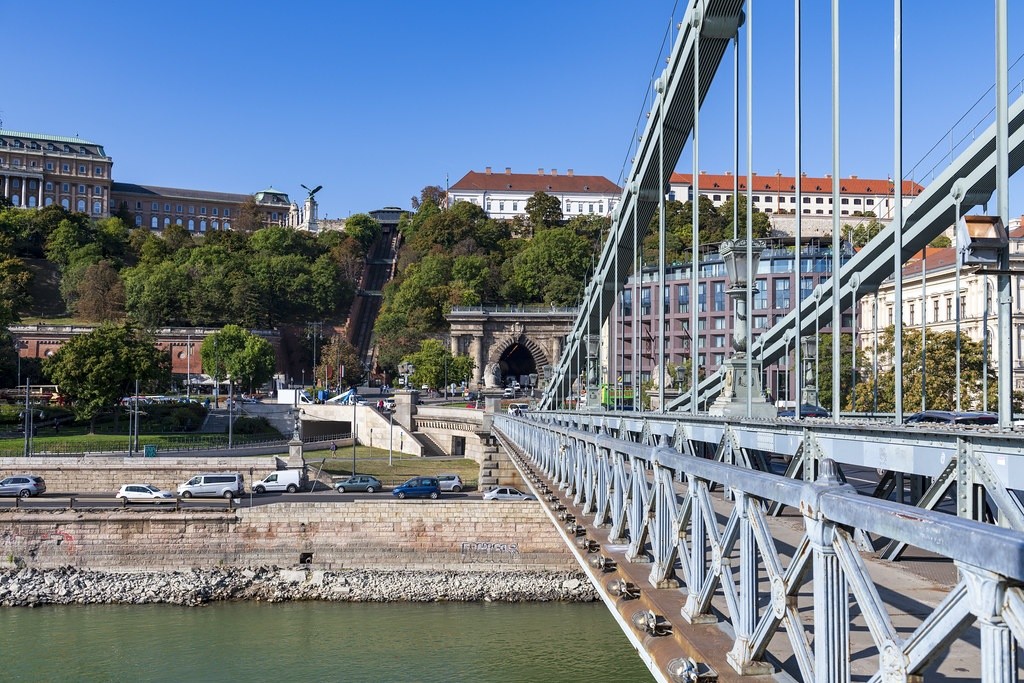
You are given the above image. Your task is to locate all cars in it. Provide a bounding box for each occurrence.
[349,394,371,406]
[508,402,530,416]
[483,486,537,500]
[392,476,441,499]
[334,475,383,493]
[423,474,464,492]
[382,398,396,411]
[778,405,828,418]
[500,374,539,398]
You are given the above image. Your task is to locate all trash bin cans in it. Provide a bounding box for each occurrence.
[144,444,157,457]
[317,390,328,401]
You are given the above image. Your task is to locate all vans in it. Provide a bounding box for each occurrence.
[177,474,246,498]
[250,469,302,493]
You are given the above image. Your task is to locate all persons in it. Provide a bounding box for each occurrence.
[180,369,593,414]
[329,441,338,459]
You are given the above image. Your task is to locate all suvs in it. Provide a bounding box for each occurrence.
[0,475,47,498]
[116,483,173,505]
[877,411,999,478]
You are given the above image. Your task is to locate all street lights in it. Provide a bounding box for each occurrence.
[122,397,153,452]
[20,408,46,456]
